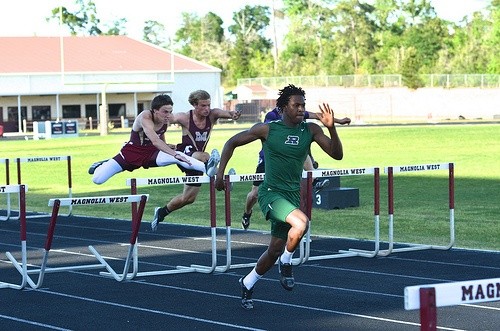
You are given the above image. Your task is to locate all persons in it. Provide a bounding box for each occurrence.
[242,107,351,231]
[214,84,343,310]
[151,90,241,231]
[88,94,221,185]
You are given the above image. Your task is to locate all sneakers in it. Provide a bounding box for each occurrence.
[278,259,296,291]
[204,148,221,179]
[88,158,110,174]
[240,278,255,312]
[227,168,236,191]
[241,208,253,231]
[152,206,166,233]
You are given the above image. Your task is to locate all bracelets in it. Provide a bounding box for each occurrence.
[174,153,181,159]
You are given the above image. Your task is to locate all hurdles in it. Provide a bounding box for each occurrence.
[404,278,500,331]
[191,171,304,273]
[99,175,217,280]
[0,155,73,221]
[292,167,380,264]
[348,162,455,256]
[5,191,150,289]
[0,184,29,289]
